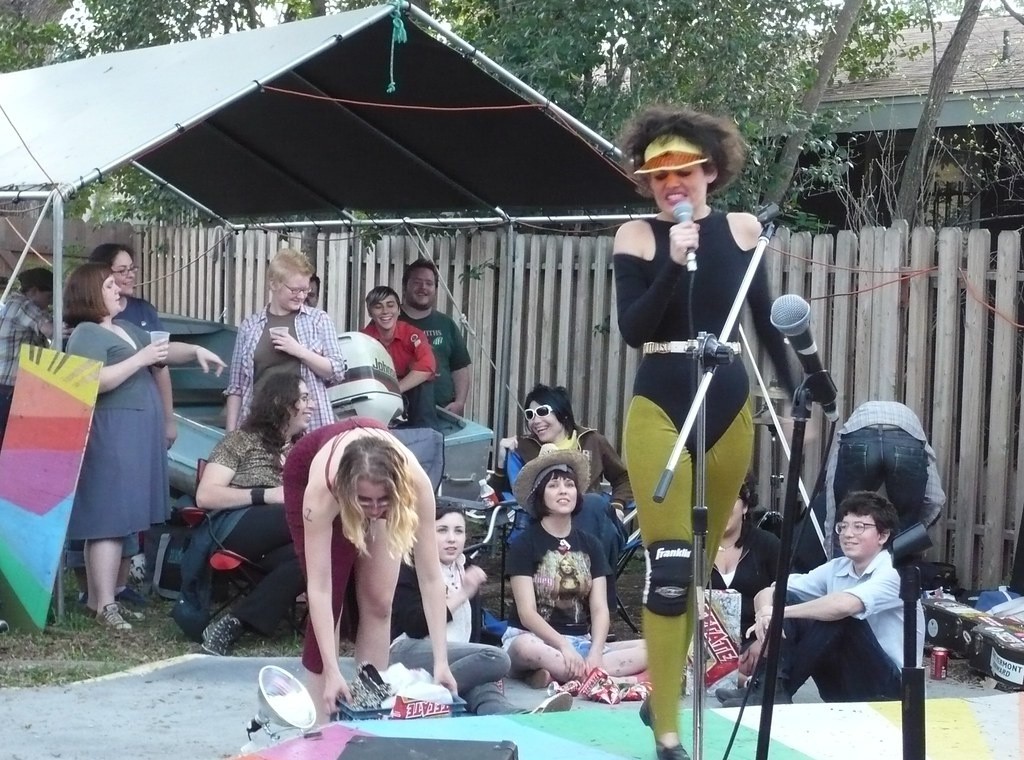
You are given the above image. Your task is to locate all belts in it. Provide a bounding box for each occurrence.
[866,425,900,431]
[643,340,742,355]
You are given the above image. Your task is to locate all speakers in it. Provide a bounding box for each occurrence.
[335,735,520,760]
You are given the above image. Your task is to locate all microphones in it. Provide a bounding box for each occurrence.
[672,200,697,273]
[770,294,840,424]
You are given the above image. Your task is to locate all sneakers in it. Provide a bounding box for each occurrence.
[715,671,792,707]
[201,613,243,657]
[94,603,133,632]
[85,601,146,622]
[115,585,146,605]
[78,592,88,602]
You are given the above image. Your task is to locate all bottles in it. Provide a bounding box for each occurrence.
[479,479,499,507]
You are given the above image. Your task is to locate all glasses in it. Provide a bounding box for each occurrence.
[112,266,138,276]
[358,499,391,508]
[835,521,876,535]
[283,282,313,295]
[436,498,467,515]
[524,404,554,421]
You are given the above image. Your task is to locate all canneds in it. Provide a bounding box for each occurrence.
[931,647,949,679]
[548,667,642,705]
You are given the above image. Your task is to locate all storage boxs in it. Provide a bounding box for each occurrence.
[683,589,742,694]
[331,681,467,723]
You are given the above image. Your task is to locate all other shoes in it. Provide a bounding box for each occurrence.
[529,692,573,713]
[525,669,555,689]
[655,740,690,760]
[638,698,655,732]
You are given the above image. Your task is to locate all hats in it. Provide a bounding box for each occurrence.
[512,444,592,519]
[634,134,712,174]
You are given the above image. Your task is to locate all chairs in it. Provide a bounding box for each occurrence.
[501,446,643,634]
[180,457,308,640]
[388,428,509,527]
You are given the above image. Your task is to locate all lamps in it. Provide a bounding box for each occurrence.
[246,664,317,740]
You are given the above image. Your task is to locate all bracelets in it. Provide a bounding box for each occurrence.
[611,503,623,508]
[251,488,265,506]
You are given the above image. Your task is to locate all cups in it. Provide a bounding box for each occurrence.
[269,326,289,349]
[150,331,170,352]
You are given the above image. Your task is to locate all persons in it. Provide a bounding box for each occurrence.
[64,261,227,631]
[388,507,573,716]
[283,417,458,726]
[0,268,53,452]
[361,286,439,429]
[221,249,345,436]
[716,491,904,708]
[196,372,315,656]
[397,259,472,416]
[517,383,634,641]
[66,242,178,605]
[503,443,648,689]
[705,484,781,688]
[611,108,798,760]
[823,401,946,563]
